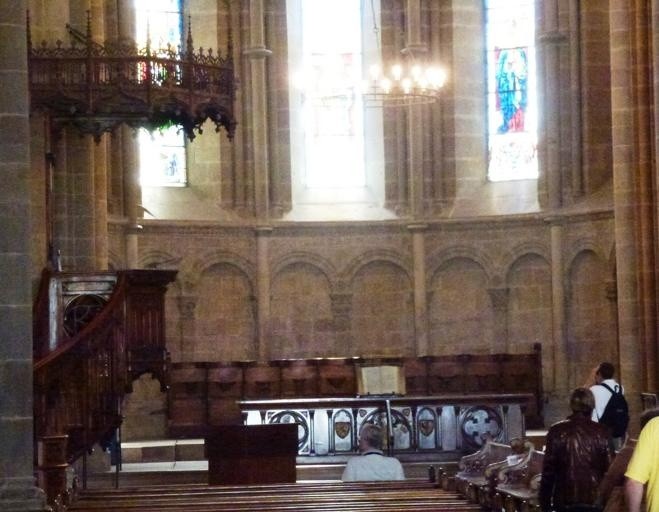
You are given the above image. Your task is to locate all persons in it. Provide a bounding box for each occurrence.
[538,387,616,512]
[341,424,406,480]
[640,408,659,429]
[583,362,628,454]
[623,416,659,512]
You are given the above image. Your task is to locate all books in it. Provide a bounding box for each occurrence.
[356,364,407,395]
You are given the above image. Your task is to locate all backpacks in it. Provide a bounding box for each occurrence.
[598,385,630,440]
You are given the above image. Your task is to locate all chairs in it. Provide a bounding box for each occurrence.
[456,434,545,509]
